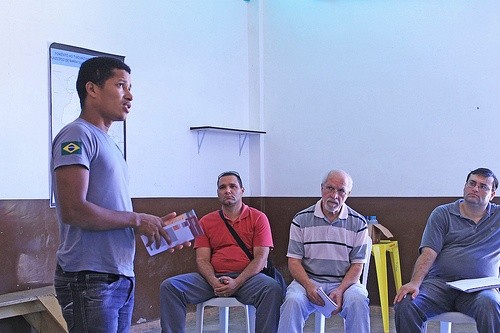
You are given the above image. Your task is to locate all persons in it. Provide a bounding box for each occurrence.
[160,171,283,333]
[50,57,191,333]
[278,170,371,333]
[394,168,500,333]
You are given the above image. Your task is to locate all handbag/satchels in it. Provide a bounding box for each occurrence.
[260,256,276,279]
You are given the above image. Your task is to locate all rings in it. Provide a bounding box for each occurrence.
[223,293,225,297]
[219,277,221,281]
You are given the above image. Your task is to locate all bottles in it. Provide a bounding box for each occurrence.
[364,216,369,225]
[369,216,378,224]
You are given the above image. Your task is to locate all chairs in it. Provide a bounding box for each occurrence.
[313,237,372,333]
[196,295,256,333]
[421,310,478,333]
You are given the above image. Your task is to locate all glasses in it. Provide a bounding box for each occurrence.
[218,171,240,179]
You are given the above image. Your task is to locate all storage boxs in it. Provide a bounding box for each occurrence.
[369,224,393,243]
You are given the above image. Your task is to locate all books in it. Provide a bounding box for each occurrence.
[310,287,339,319]
[445,277,500,293]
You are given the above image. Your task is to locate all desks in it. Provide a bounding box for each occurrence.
[371,240,403,333]
